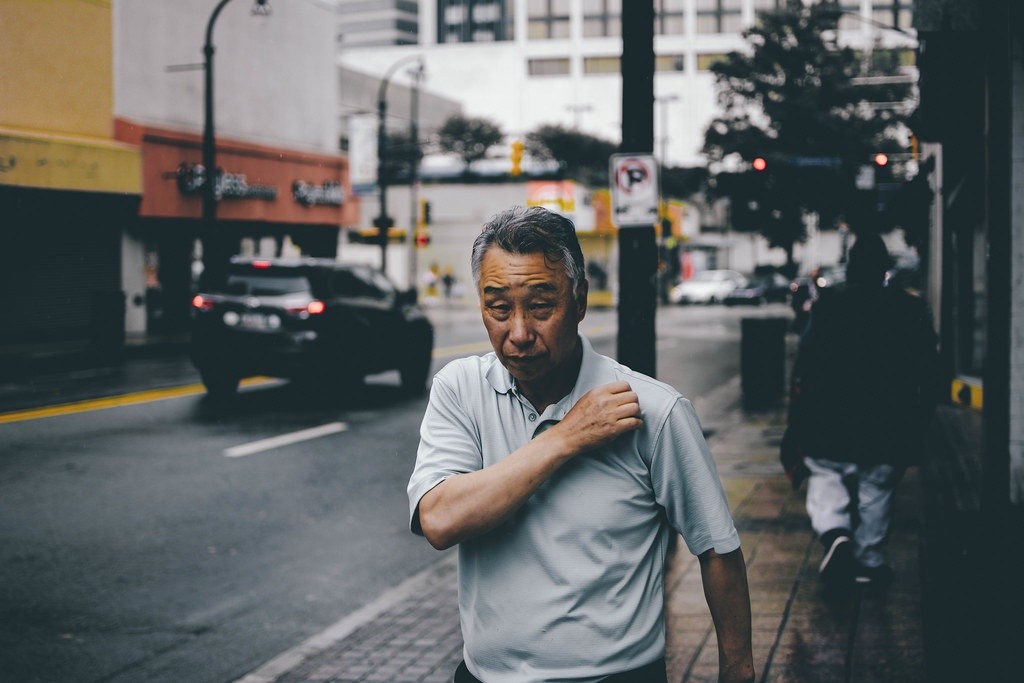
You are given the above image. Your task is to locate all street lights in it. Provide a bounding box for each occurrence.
[201,0,274,270]
[375,52,426,270]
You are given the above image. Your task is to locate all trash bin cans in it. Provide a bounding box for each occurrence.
[740,314,786,406]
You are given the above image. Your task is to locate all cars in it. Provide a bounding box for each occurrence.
[729,264,846,306]
[667,269,749,306]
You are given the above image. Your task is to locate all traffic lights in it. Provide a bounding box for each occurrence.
[875,153,888,166]
[753,156,766,171]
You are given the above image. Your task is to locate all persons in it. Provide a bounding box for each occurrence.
[782,242,941,583]
[407,206,756,683]
[792,266,821,333]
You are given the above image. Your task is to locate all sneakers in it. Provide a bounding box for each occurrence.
[818,526,854,579]
[853,568,873,584]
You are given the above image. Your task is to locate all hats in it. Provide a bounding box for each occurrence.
[848,234,896,272]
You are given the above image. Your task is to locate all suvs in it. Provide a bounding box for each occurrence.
[183,256,437,415]
